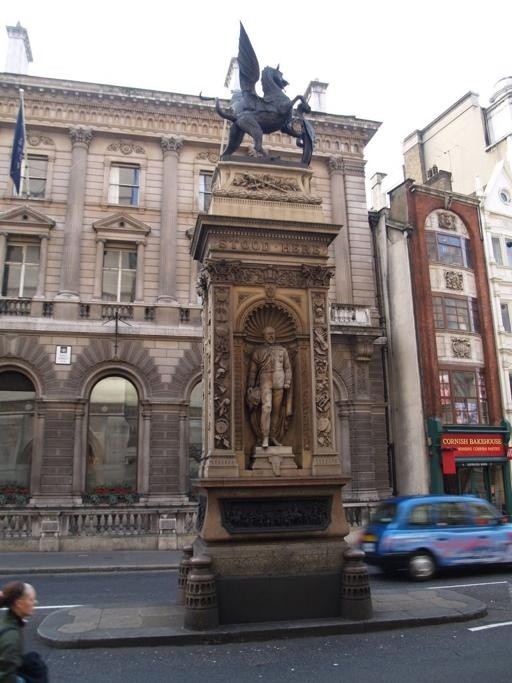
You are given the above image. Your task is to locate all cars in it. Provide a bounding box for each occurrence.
[358,492,512,583]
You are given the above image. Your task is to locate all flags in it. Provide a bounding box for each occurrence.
[10,100,24,193]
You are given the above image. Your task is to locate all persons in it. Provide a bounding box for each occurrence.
[249,325,293,448]
[0,580,49,683]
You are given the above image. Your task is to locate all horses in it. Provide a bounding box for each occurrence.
[199,61,311,158]
